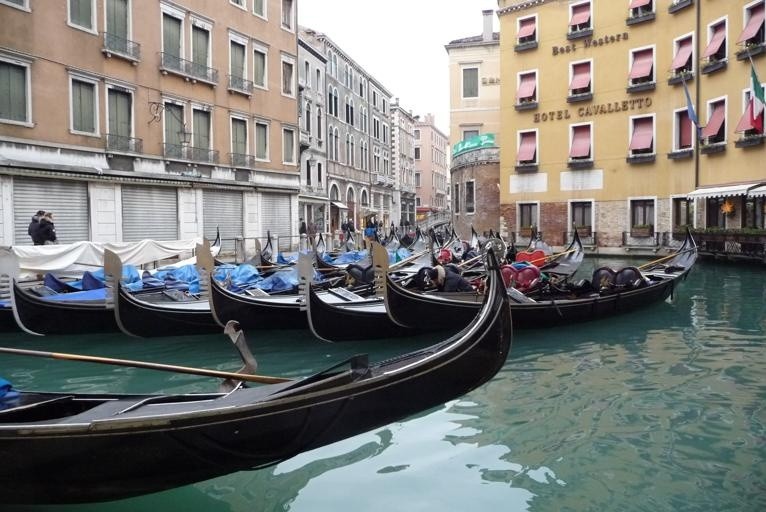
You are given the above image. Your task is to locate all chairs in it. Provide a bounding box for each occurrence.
[347,240,642,297]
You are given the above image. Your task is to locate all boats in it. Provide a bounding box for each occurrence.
[0,248,515,508]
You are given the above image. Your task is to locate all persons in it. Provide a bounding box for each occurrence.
[300,213,410,247]
[38,213,56,245]
[415,266,474,292]
[28,210,45,245]
